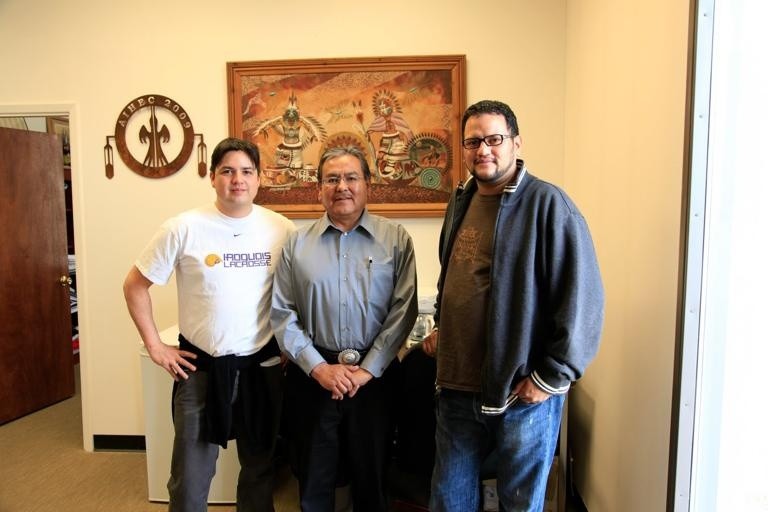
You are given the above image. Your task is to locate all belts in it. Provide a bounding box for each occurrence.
[313,346,368,365]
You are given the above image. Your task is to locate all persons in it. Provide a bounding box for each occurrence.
[269,145,419,508]
[122,135,294,512]
[421,100,604,511]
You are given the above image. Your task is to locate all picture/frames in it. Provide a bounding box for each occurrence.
[224,52,468,221]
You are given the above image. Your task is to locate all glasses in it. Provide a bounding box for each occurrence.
[462,134,515,149]
